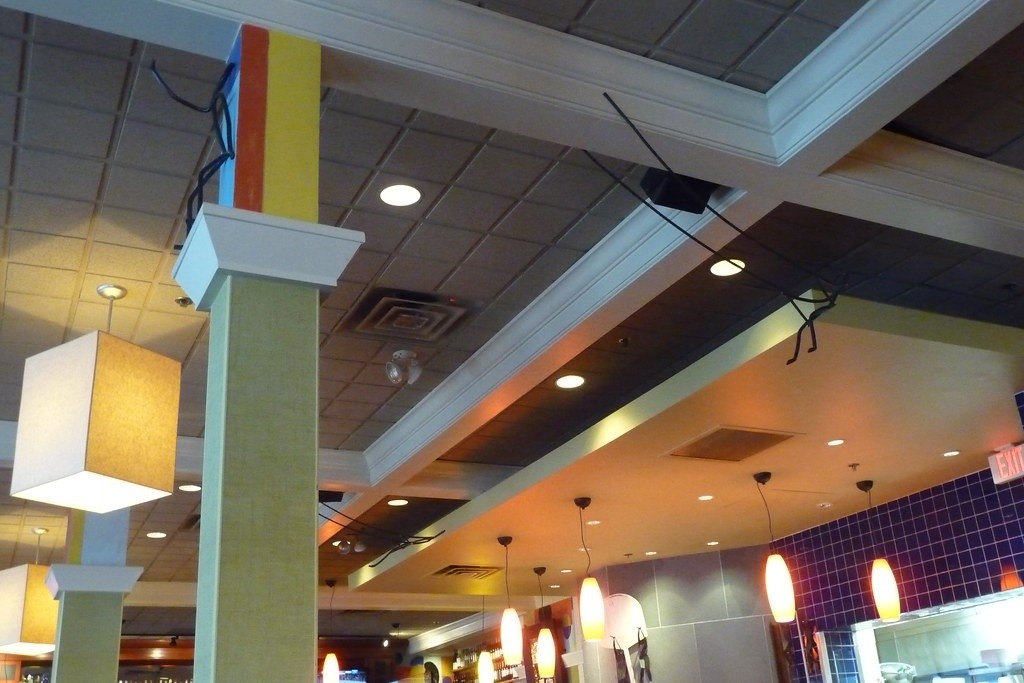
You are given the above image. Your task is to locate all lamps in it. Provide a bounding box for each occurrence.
[497,535,523,665]
[856,480,900,623]
[533,566,556,678]
[574,496,605,642]
[7,285,182,514]
[477,595,495,683]
[323,579,340,683]
[752,471,795,623]
[0,528,60,656]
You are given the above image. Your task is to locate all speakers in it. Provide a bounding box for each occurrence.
[640,168,719,215]
